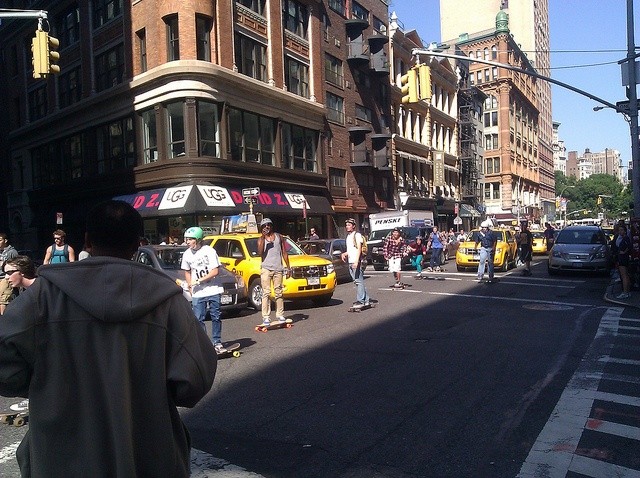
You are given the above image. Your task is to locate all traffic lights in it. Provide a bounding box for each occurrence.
[31,30,60,79]
[396,64,433,104]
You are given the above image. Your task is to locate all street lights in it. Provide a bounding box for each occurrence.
[516,166,538,226]
[559,185,575,220]
[592,103,632,128]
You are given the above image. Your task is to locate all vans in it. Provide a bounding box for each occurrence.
[455,227,518,272]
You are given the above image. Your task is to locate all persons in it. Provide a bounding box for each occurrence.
[543,224,556,253]
[383,226,406,288]
[518,220,534,276]
[426,226,446,271]
[440,227,468,245]
[341,217,370,308]
[609,219,633,299]
[307,228,319,239]
[406,235,426,279]
[255,218,291,323]
[474,221,497,282]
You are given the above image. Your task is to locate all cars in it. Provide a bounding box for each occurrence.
[531,230,548,254]
[548,225,609,273]
[137,245,240,316]
[296,239,368,284]
[443,234,460,260]
[203,233,337,311]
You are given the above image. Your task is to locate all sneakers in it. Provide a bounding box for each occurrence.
[214,344,227,352]
[263,317,271,325]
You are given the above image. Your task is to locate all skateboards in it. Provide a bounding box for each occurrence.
[393,285,407,291]
[349,302,376,312]
[472,276,498,285]
[435,268,448,273]
[0,408,30,427]
[256,318,292,332]
[215,343,240,358]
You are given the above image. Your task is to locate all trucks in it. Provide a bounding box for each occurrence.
[365,209,436,271]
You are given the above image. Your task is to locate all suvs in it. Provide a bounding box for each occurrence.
[384,225,445,270]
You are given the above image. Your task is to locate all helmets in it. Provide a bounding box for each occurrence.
[183,227,203,243]
[260,218,274,228]
[345,218,356,224]
